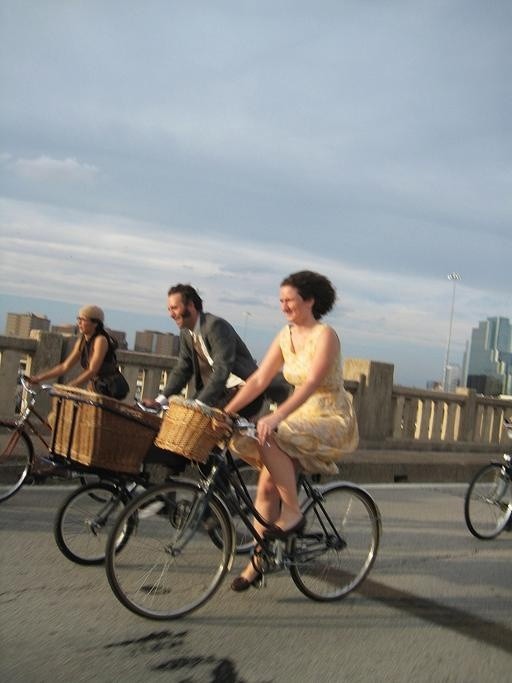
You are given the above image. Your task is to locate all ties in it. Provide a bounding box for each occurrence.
[191,335,214,388]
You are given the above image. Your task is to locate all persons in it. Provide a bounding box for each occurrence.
[138,284,265,530]
[221,270,360,593]
[30,304,129,466]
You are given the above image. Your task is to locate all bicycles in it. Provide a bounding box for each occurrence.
[465,417,512,539]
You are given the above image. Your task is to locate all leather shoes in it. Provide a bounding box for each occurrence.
[230,568,263,593]
[262,512,307,541]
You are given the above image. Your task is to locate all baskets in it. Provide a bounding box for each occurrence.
[49,384,163,476]
[153,393,233,464]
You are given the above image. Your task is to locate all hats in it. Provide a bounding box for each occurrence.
[80,305,103,320]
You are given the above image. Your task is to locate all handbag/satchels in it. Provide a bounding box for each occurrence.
[92,371,129,400]
